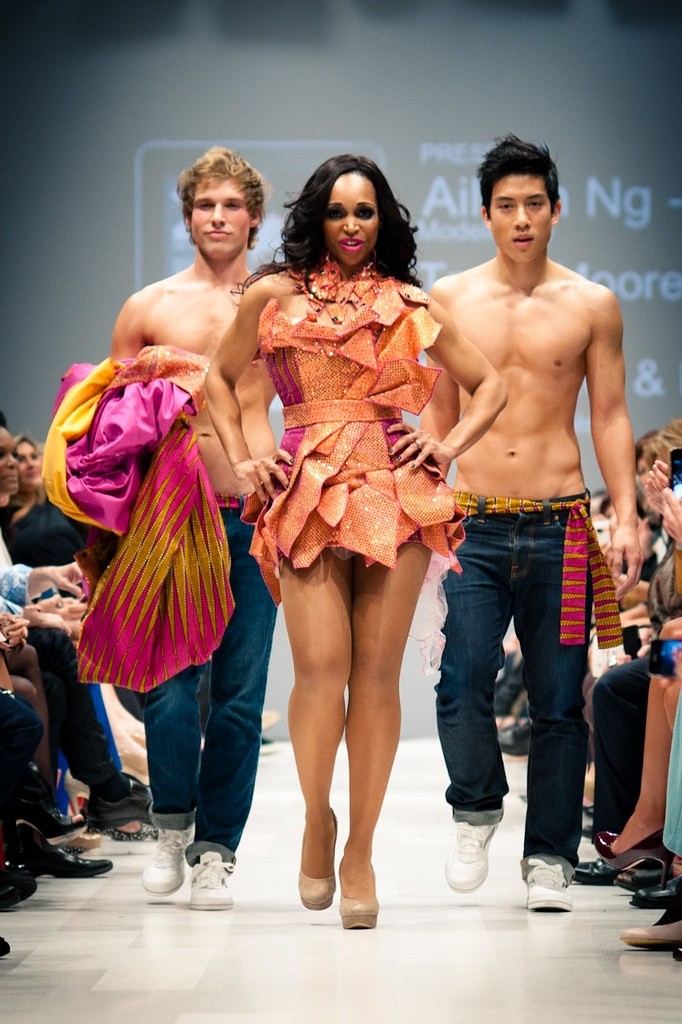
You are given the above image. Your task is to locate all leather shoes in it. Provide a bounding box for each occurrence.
[0,867,36,905]
[85,772,154,828]
[23,844,114,879]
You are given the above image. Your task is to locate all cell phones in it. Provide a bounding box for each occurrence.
[648,639,682,678]
[669,448,682,500]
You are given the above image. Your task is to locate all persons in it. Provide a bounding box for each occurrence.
[496,417,682,961]
[205,155,507,931]
[0,409,281,958]
[112,147,278,909]
[420,134,644,910]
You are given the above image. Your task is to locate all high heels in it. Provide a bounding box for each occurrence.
[296,806,338,909]
[85,819,160,842]
[10,797,84,838]
[337,857,378,930]
[594,826,675,889]
[59,843,86,855]
[64,768,94,817]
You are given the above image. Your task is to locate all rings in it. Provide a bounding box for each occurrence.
[415,439,421,449]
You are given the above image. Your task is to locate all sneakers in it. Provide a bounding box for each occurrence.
[141,828,191,896]
[189,850,235,911]
[444,823,498,894]
[525,858,573,913]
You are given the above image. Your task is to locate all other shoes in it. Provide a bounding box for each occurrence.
[499,722,531,755]
[573,856,682,960]
